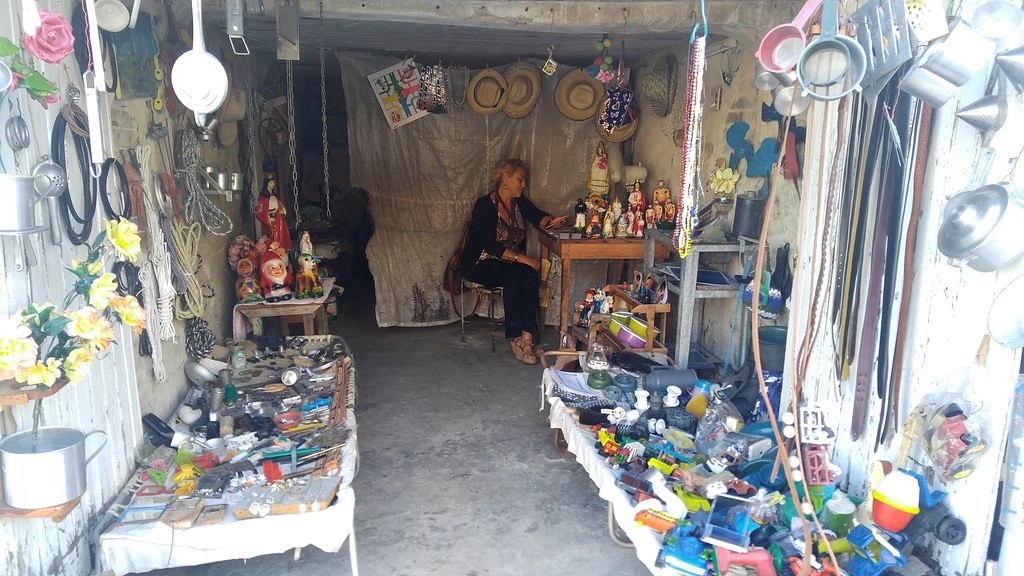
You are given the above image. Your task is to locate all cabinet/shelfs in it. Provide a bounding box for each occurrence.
[641,226,767,376]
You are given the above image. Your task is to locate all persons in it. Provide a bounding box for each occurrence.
[255,171,291,248]
[586,140,610,197]
[574,195,645,238]
[260,251,293,302]
[653,199,663,223]
[645,204,655,229]
[654,179,670,208]
[460,159,570,365]
[628,179,647,213]
[664,198,675,223]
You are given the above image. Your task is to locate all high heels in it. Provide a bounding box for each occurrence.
[511,340,543,358]
[516,339,536,364]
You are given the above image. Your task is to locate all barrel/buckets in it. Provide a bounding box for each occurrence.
[748,325,787,373]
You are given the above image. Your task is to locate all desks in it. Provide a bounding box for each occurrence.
[231,275,335,338]
[532,221,673,355]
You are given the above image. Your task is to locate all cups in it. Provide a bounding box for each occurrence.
[641,364,700,395]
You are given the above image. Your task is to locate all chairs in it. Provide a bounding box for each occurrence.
[455,220,504,355]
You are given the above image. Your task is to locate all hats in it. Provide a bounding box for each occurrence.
[596,88,639,142]
[553,68,605,120]
[465,67,508,115]
[501,62,543,119]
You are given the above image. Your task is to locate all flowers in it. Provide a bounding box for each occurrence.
[0,216,148,453]
[0,7,77,111]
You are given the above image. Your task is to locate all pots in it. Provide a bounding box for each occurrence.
[913,18,997,87]
[936,179,1024,273]
[229,173,245,190]
[209,171,229,190]
[897,59,956,110]
[0,425,109,509]
[200,166,218,190]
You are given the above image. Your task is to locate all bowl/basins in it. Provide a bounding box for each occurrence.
[617,326,646,348]
[609,319,628,334]
[629,315,661,341]
[611,311,633,325]
